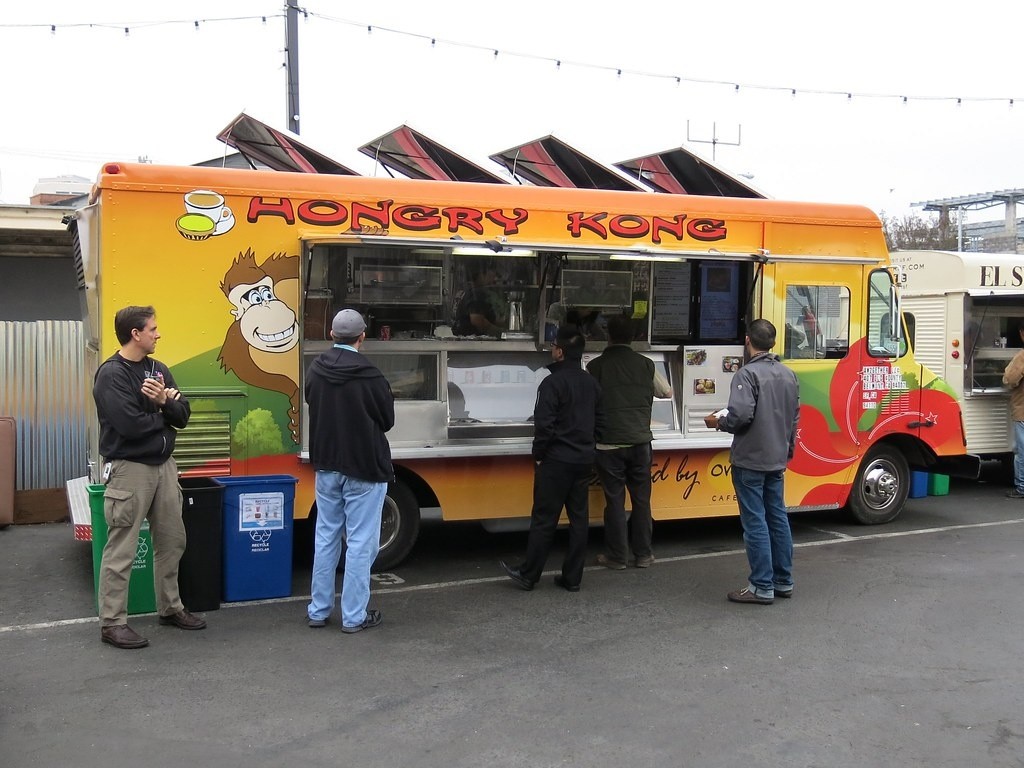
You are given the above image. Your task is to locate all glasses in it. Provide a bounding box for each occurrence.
[550,341,559,348]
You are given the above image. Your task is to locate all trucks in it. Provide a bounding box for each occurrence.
[842,249,1024,477]
[87,161,981,572]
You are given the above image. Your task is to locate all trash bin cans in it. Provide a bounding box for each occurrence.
[212,474,299,601]
[176,476,226,612]
[84,484,157,615]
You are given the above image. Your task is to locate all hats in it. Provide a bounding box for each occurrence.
[331,308,367,339]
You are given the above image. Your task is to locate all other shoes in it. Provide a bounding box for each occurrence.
[1005,489,1024,498]
[341,609,381,633]
[727,587,774,605]
[159,608,207,629]
[500,561,534,591]
[553,574,580,591]
[773,589,793,598]
[635,554,655,567]
[304,614,332,626]
[101,624,150,649]
[597,554,626,569]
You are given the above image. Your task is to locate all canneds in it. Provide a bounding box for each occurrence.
[381,325,390,342]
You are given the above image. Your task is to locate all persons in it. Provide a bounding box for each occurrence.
[454,255,642,342]
[305,309,395,633]
[704,319,799,604]
[585,315,672,571]
[92,305,206,649]
[1003,325,1024,499]
[501,328,606,592]
